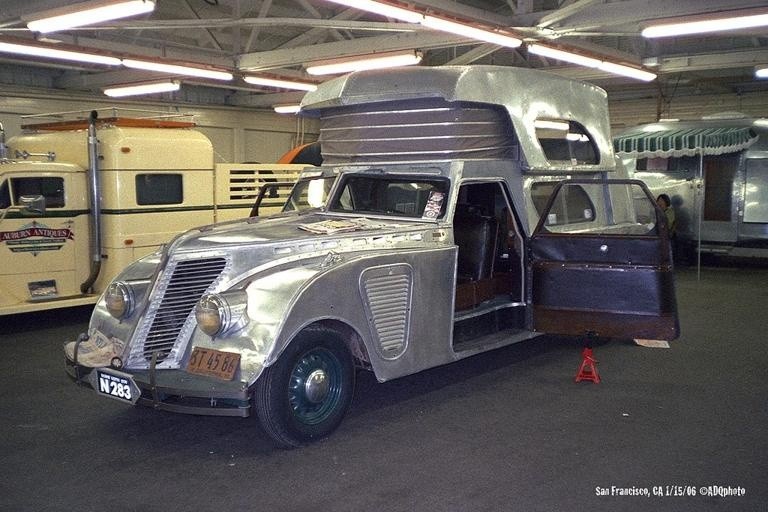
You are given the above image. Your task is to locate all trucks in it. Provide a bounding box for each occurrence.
[0,106,334,324]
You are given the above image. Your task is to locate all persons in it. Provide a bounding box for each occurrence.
[650,194,676,238]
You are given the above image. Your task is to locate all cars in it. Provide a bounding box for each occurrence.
[61,62,684,452]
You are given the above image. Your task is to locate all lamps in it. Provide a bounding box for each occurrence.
[1,0,768,116]
